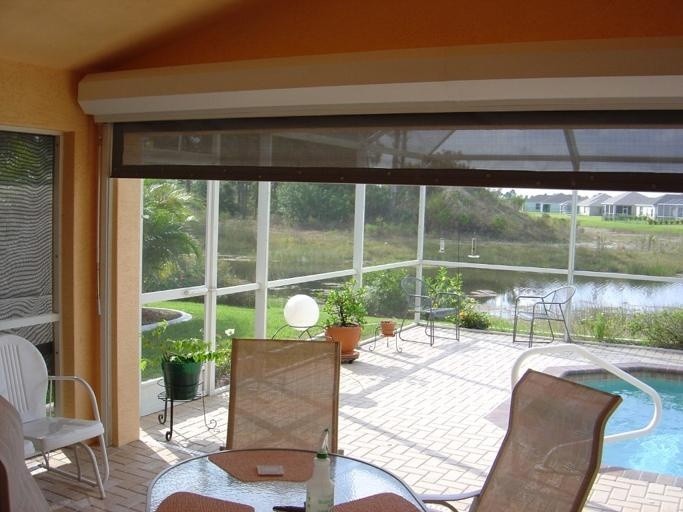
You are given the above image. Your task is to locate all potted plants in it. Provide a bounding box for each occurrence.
[138,318,233,401]
[380,319,395,336]
[323,278,368,354]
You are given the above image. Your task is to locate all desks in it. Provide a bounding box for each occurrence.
[146,449,428,511]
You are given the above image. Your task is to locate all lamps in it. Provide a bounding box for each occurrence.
[271,292,320,340]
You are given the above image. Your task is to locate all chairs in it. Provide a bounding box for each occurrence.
[416,368,623,512]
[220,337,344,457]
[512,286,576,348]
[399,273,460,347]
[0,333,110,512]
